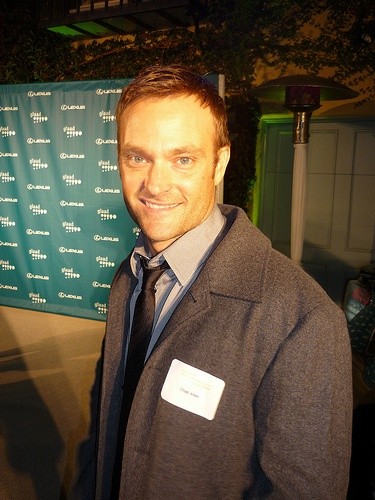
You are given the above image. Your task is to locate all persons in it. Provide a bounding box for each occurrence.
[94,63,353,500]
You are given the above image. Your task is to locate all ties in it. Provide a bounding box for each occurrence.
[111,256,171,500]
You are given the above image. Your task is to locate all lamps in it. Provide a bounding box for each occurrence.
[251,73,359,143]
